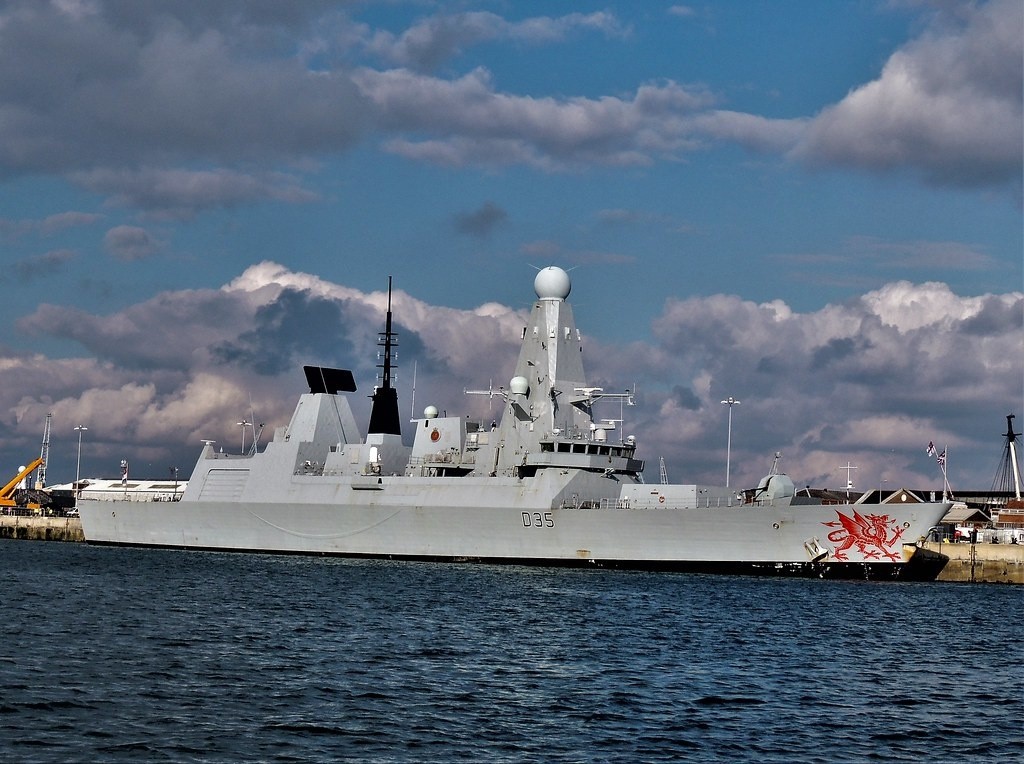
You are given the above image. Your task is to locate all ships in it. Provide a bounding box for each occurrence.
[74,263,957,586]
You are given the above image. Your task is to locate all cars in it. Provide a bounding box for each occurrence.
[66,507,79,518]
